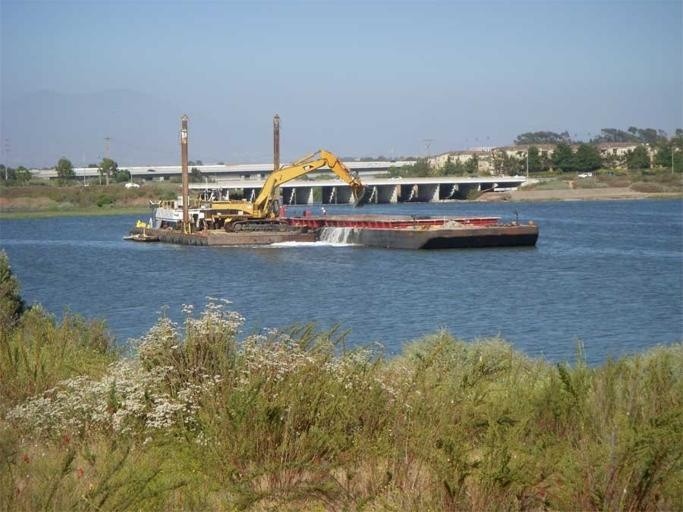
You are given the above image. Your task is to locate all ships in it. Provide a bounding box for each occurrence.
[123,113,321,245]
[288,216,539,249]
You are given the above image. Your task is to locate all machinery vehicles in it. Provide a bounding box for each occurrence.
[204,150,364,232]
